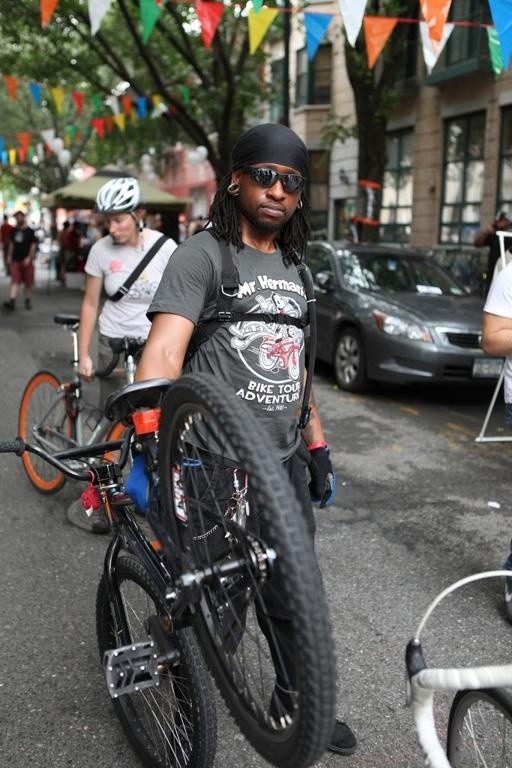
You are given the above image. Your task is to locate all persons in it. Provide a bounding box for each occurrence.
[475,217,512,296]
[77,176,177,418]
[59,221,71,286]
[3,211,39,310]
[135,124,357,756]
[73,219,81,250]
[478,256,511,618]
[0,215,15,275]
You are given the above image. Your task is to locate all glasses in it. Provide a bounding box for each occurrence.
[240,166,305,192]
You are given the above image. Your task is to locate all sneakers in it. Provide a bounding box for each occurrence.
[266,704,357,755]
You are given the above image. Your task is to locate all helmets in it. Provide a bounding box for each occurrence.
[96,177,140,214]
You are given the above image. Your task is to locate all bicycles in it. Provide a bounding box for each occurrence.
[49,380,335,760]
[7,311,178,502]
[446,687,512,767]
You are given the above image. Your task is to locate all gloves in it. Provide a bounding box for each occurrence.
[308,446,337,508]
[125,455,160,517]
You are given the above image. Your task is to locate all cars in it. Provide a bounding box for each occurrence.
[292,240,496,397]
